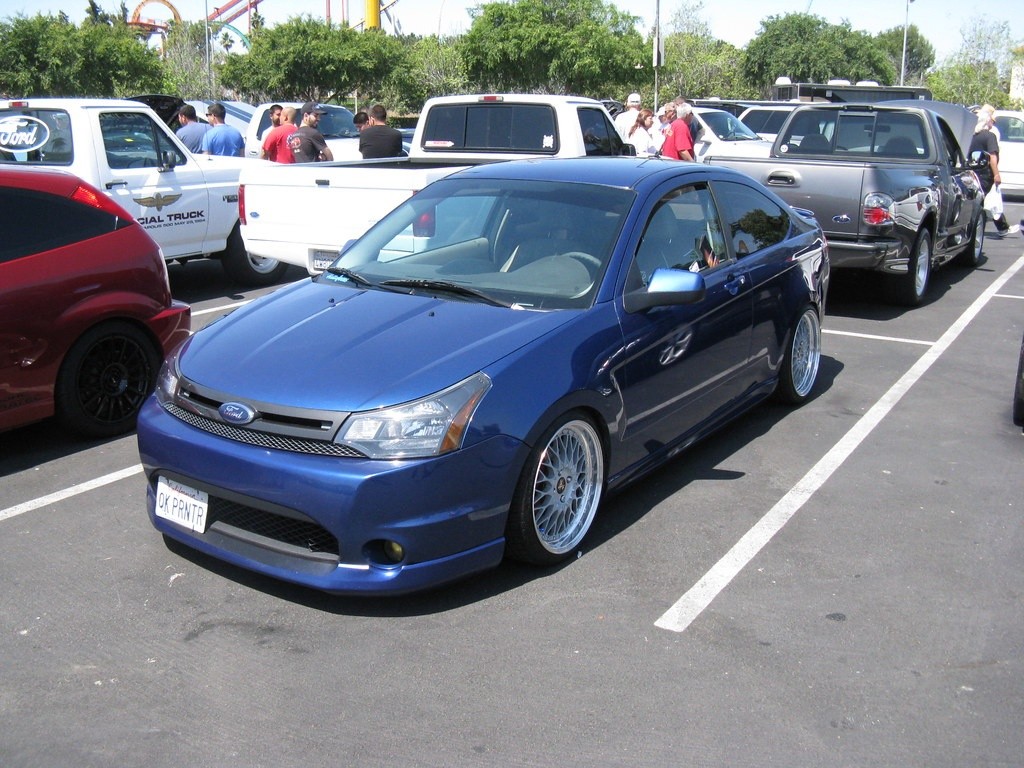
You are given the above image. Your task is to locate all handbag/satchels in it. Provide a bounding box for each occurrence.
[983,183,1003,220]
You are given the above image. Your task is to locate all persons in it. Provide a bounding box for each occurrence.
[966,103,1019,236]
[202,103,246,157]
[353,112,372,153]
[359,104,402,159]
[176,104,213,154]
[615,92,695,162]
[287,101,334,163]
[260,104,297,160]
[261,106,299,163]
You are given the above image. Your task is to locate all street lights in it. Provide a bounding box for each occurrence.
[900,0,916,86]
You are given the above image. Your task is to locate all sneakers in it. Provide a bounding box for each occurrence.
[999,224,1020,236]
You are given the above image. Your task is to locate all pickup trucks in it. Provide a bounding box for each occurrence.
[0,94,637,286]
[684,77,1024,306]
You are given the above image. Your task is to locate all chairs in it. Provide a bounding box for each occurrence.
[883,136,918,155]
[499,199,583,273]
[635,201,680,282]
[798,133,831,154]
[565,198,618,280]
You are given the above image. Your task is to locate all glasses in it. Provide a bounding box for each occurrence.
[206,112,215,117]
[689,113,694,116]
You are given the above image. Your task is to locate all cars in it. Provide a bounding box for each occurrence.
[137,156,830,599]
[0,165,191,438]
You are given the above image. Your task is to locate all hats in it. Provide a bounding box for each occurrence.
[657,106,666,118]
[628,93,641,105]
[973,104,996,124]
[301,102,328,116]
